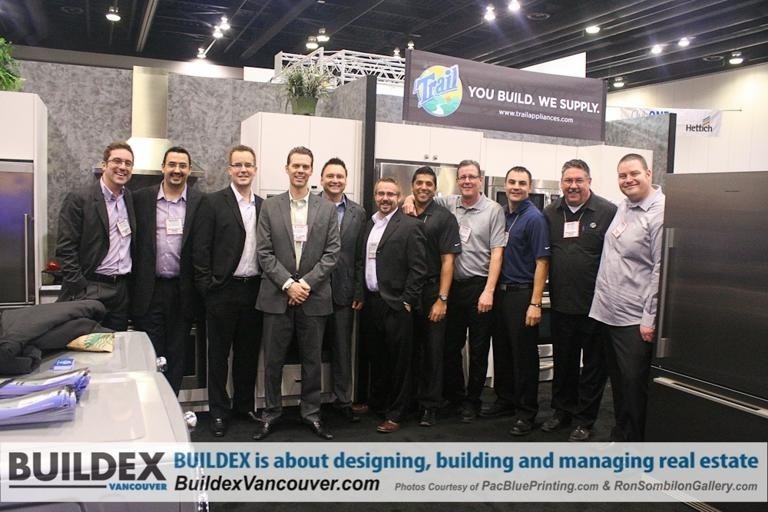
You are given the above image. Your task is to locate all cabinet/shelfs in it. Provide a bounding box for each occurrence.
[485,136,521,179]
[374,121,483,166]
[240,111,360,200]
[579,145,653,206]
[555,145,578,183]
[521,142,557,181]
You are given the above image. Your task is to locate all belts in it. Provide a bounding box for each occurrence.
[497,282,531,292]
[366,290,381,299]
[92,273,124,284]
[232,275,260,286]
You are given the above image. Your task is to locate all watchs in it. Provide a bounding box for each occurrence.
[528,300,544,309]
[437,292,448,300]
[283,280,295,291]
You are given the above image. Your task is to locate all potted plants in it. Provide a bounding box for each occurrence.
[270,64,335,116]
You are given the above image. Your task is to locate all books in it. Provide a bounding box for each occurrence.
[0,367,92,403]
[0,387,76,427]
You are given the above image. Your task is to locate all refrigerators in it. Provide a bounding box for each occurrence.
[644,170,768,446]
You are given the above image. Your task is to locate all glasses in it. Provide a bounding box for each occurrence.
[457,175,482,181]
[163,162,188,169]
[105,157,133,166]
[375,191,398,198]
[229,162,256,169]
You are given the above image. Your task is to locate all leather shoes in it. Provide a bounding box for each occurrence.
[301,416,336,440]
[509,416,536,436]
[350,400,370,414]
[239,411,265,426]
[418,406,438,427]
[459,402,478,422]
[376,419,400,433]
[212,416,225,437]
[480,403,515,418]
[252,419,277,440]
[539,418,563,433]
[567,425,593,442]
[341,406,363,423]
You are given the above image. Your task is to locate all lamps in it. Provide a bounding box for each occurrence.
[679,38,691,48]
[393,47,401,59]
[484,4,497,22]
[651,45,662,56]
[196,45,208,62]
[106,0,122,23]
[407,41,415,50]
[317,28,330,43]
[218,15,230,32]
[212,24,223,41]
[509,0,520,11]
[614,76,625,88]
[728,52,744,64]
[305,34,319,50]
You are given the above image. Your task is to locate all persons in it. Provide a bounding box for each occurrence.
[131,146,208,396]
[193,144,265,438]
[588,154,666,441]
[543,158,620,432]
[478,166,552,436]
[352,177,429,432]
[315,157,369,422]
[402,161,506,424]
[397,166,463,425]
[253,147,343,439]
[56,143,138,331]
